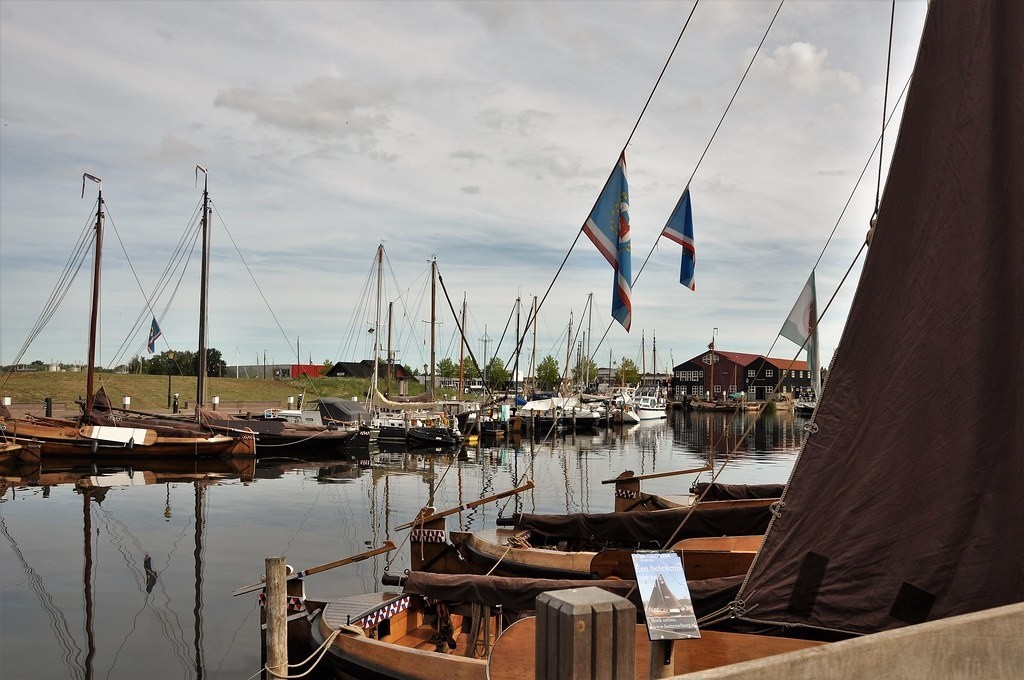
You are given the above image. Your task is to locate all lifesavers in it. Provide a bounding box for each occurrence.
[556,410,561,418]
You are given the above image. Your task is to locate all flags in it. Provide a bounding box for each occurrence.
[583,149,632,334]
[147,318,162,354]
[780,271,821,400]
[661,186,696,291]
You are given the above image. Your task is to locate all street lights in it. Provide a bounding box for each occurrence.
[423,364,429,393]
[166,349,174,410]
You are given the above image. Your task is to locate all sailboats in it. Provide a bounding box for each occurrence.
[1,0,1024,680]
